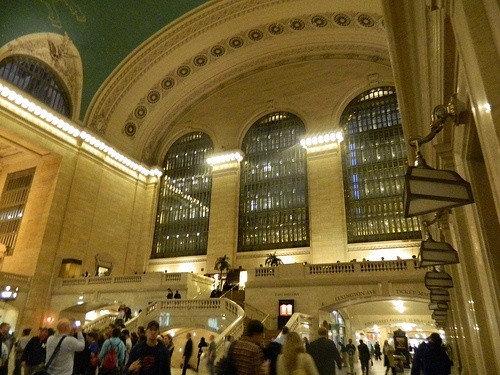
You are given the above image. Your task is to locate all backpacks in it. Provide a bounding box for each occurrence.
[216,340,239,375]
[103,338,121,369]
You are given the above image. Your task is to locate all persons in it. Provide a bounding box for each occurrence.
[345,338,356,373]
[0,323,11,375]
[80,271,100,278]
[198,337,207,371]
[370,342,382,360]
[209,335,219,375]
[12,318,174,375]
[209,289,222,298]
[382,341,397,375]
[222,320,342,375]
[337,341,348,367]
[166,288,173,300]
[410,333,454,375]
[174,290,181,299]
[183,333,196,375]
[116,305,133,318]
[358,340,371,375]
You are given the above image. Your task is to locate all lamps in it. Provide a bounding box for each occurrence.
[424,265,453,329]
[416,209,459,268]
[401,95,474,218]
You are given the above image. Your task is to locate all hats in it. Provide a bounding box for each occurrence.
[146,320,159,329]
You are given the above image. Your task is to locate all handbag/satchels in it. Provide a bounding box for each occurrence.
[31,364,50,375]
